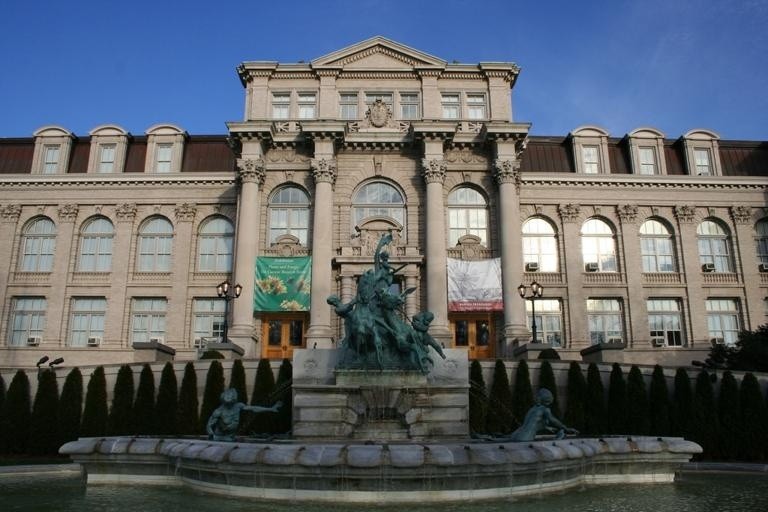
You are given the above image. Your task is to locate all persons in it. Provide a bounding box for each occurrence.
[508,387,580,442]
[326,232,446,376]
[206,386,283,441]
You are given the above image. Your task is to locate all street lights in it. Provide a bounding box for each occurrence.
[216,279,244,341]
[519,279,546,344]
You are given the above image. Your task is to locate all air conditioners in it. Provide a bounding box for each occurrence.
[150,337,163,345]
[526,262,768,346]
[27,335,41,346]
[194,336,209,346]
[87,336,100,346]
[698,171,710,176]
